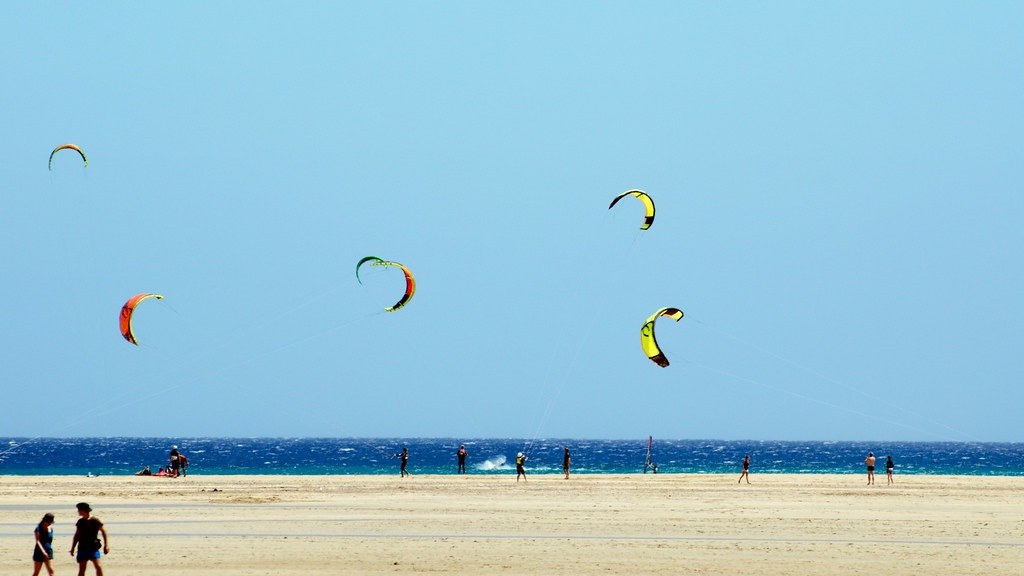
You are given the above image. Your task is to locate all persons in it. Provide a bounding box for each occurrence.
[864,451,875,486]
[140,445,189,478]
[884,455,895,486]
[515,452,529,482]
[457,445,468,475]
[71,502,110,576]
[32,513,57,576]
[562,448,571,479]
[738,456,752,484]
[393,448,410,478]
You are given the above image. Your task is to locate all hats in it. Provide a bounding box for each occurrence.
[43,512,55,523]
[517,452,523,457]
[172,445,178,449]
[76,502,93,511]
